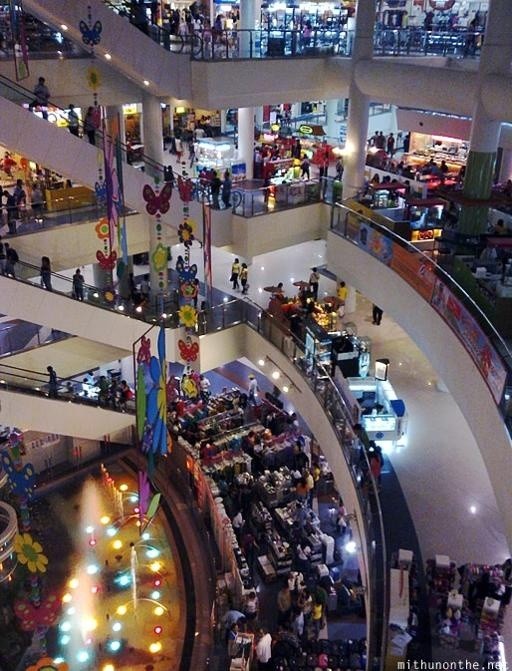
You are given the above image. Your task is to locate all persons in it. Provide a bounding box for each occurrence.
[198,373,211,401]
[3,242,20,279]
[66,370,129,412]
[3,189,16,235]
[277,283,283,292]
[0,235,3,256]
[40,256,52,290]
[247,374,261,407]
[171,419,384,671]
[193,279,199,307]
[18,190,26,217]
[480,240,497,259]
[188,137,196,169]
[318,153,329,182]
[140,274,151,300]
[337,281,348,318]
[29,76,51,120]
[214,15,222,30]
[222,171,232,209]
[72,268,85,301]
[47,366,58,398]
[0,151,10,166]
[177,17,188,36]
[67,104,79,137]
[240,263,248,294]
[372,303,383,326]
[231,16,237,38]
[369,129,466,184]
[309,267,320,301]
[31,183,43,218]
[200,167,207,177]
[494,219,507,235]
[126,272,137,300]
[230,393,247,410]
[83,107,96,145]
[253,141,281,179]
[3,155,17,177]
[13,179,22,219]
[284,104,291,124]
[333,156,344,181]
[231,257,241,290]
[193,124,207,138]
[203,19,211,42]
[263,170,272,202]
[174,134,183,163]
[303,20,312,48]
[300,154,310,180]
[190,13,201,31]
[274,105,283,125]
[210,172,221,210]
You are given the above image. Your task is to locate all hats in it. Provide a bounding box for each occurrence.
[248,374,255,379]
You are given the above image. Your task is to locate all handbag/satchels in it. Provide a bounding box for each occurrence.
[338,517,347,528]
[230,276,234,281]
[244,280,249,289]
